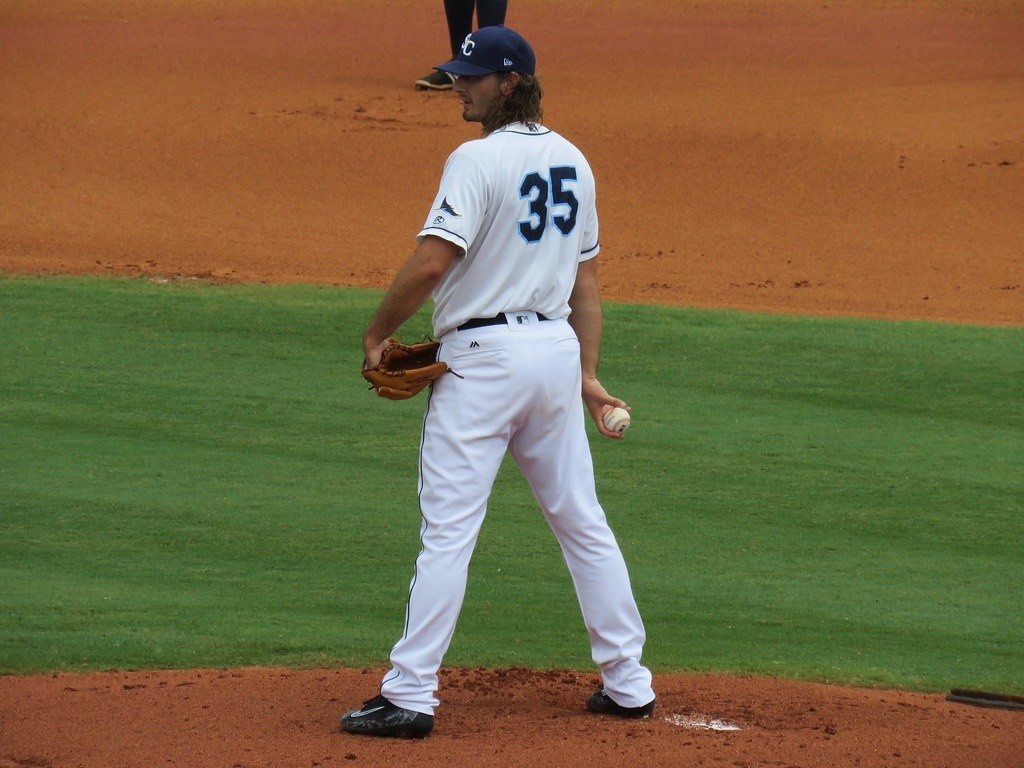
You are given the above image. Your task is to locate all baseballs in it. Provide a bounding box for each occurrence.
[603,407,632,435]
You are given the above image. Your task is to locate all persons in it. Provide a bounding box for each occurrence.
[418,0,506,91]
[336,27,659,738]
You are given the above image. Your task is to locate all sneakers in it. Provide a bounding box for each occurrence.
[340,694,433,739]
[414,70,460,90]
[586,688,655,718]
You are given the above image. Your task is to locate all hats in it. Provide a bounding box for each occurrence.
[430,25,535,76]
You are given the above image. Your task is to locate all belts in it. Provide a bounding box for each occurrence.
[457,309,546,332]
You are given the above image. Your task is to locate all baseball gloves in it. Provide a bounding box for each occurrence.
[361,332,448,401]
